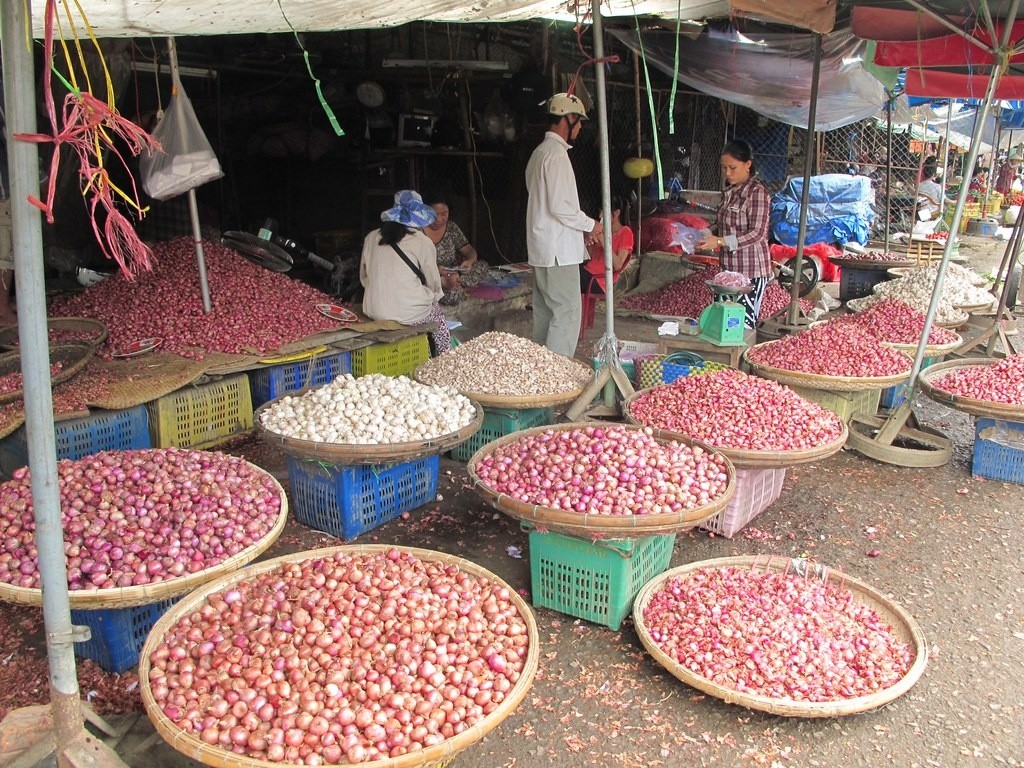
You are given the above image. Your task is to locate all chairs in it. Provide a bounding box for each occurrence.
[906,187,941,219]
[579,253,633,340]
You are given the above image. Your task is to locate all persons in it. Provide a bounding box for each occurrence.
[421,191,477,274]
[580,194,634,295]
[121,92,193,245]
[525,93,603,357]
[915,156,936,184]
[919,166,957,211]
[694,138,772,329]
[996,154,1024,197]
[861,153,895,196]
[360,190,451,358]
[970,167,990,192]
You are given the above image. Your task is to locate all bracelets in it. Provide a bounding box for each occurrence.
[717,237,722,247]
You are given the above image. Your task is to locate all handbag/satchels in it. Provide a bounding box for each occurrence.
[712,271,748,288]
[139,67,225,201]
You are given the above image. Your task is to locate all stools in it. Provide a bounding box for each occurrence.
[660,327,757,370]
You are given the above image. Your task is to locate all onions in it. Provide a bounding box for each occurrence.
[0,233,351,589]
[148,548,529,766]
[477,250,958,705]
[930,349,1024,404]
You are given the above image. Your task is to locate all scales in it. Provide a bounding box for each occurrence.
[696,280,754,347]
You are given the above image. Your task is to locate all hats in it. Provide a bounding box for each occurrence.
[382,190,437,229]
[1010,153,1022,159]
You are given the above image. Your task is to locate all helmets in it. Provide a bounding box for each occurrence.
[546,93,590,120]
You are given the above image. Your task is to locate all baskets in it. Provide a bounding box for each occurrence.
[943,192,1003,234]
[0,250,1024,768]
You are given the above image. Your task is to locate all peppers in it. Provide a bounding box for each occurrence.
[926,230,950,240]
[945,182,1024,207]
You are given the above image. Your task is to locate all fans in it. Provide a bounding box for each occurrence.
[221,218,361,294]
[626,188,658,220]
[680,249,818,297]
[335,68,412,128]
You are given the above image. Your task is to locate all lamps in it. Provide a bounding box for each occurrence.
[382,59,509,69]
[131,62,217,78]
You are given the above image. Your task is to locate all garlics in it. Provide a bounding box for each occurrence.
[418,330,592,396]
[851,258,992,324]
[260,371,478,446]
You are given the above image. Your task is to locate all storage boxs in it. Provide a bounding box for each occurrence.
[943,194,1004,234]
[6,267,939,675]
[971,416,1024,486]
